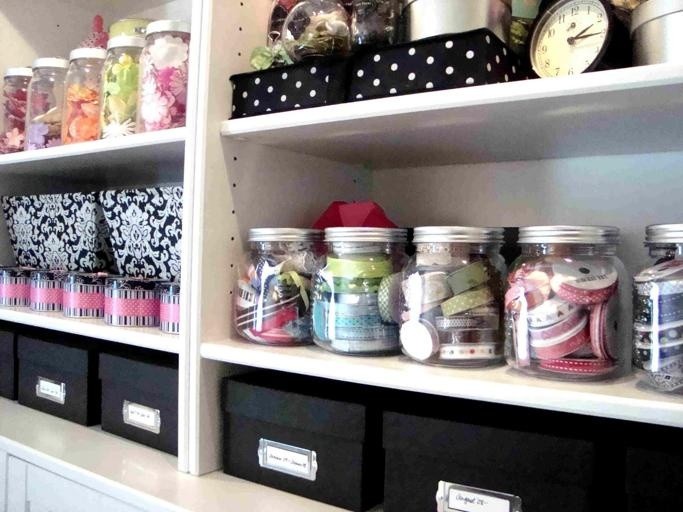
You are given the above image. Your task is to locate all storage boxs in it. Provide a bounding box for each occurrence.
[0,330,179,457]
[370,405,632,512]
[98,183,182,282]
[220,376,384,510]
[2,192,117,272]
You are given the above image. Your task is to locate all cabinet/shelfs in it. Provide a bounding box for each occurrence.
[0,1,202,474]
[189,0,683,477]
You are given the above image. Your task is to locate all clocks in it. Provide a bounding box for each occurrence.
[529,0,631,78]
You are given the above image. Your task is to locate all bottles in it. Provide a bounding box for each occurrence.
[309,221,413,358]
[24,57,70,149]
[398,225,505,368]
[137,19,191,134]
[0,67,34,155]
[630,222,683,397]
[61,47,107,144]
[232,226,323,347]
[500,224,632,386]
[98,36,145,138]
